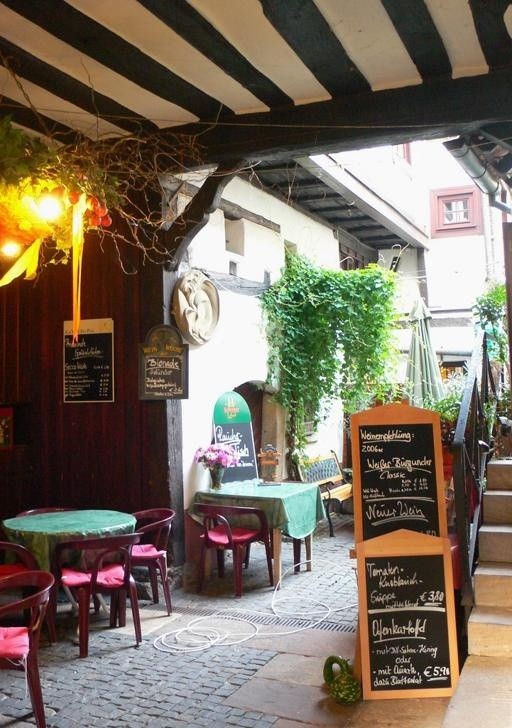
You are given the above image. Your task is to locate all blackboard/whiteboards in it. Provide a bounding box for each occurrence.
[350,403,457,702]
[65,322,116,405]
[215,422,257,482]
[141,327,191,399]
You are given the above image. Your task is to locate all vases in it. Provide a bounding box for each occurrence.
[209,464,224,490]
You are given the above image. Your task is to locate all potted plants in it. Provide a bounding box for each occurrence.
[422,391,501,479]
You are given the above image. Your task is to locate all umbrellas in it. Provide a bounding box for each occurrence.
[402,297,447,409]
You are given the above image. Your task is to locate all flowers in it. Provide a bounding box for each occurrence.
[195,443,240,469]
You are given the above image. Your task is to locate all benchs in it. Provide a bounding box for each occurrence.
[292,451,352,537]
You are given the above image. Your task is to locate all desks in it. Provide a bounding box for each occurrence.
[197,480,326,589]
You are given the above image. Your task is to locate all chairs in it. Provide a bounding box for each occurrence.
[0,506,175,728]
[193,503,273,598]
